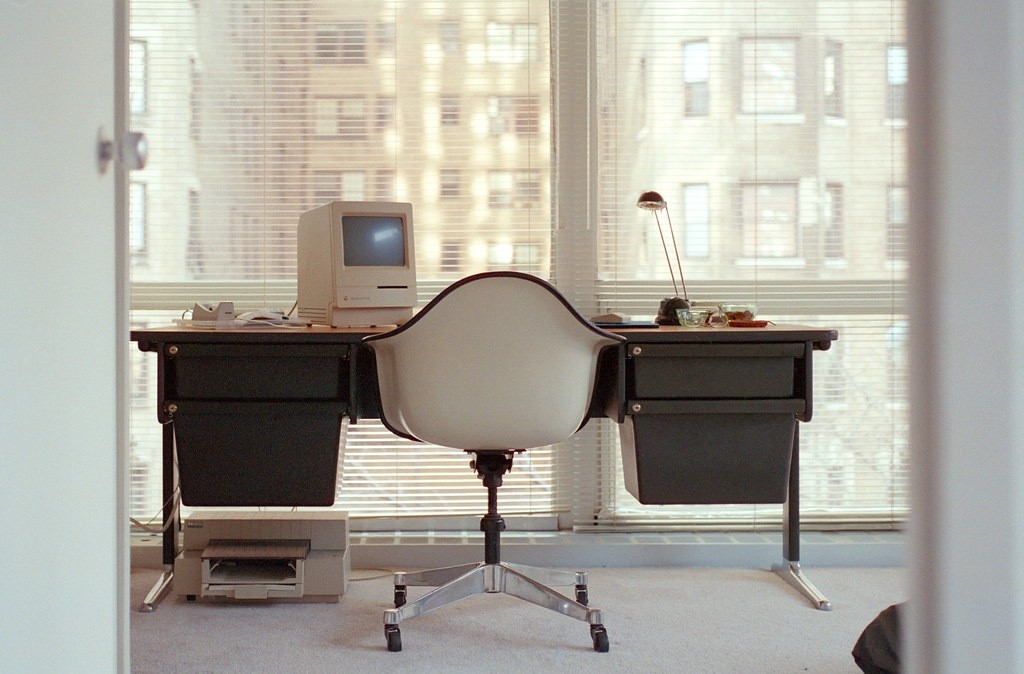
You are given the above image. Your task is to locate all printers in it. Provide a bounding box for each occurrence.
[173,511,349,604]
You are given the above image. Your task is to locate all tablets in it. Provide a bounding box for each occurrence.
[595,321,660,328]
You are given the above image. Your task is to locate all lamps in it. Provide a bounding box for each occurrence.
[637,192,691,325]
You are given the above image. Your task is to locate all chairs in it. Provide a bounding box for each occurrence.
[361,270,627,653]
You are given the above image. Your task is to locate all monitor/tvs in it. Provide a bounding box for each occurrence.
[296,200,417,328]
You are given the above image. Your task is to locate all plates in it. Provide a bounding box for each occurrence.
[729,319,768,327]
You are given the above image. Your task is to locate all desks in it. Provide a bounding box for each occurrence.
[130,324,839,613]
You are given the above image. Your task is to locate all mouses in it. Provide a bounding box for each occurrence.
[235,311,283,327]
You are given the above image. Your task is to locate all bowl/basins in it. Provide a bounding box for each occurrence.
[677,308,709,327]
[720,305,759,320]
